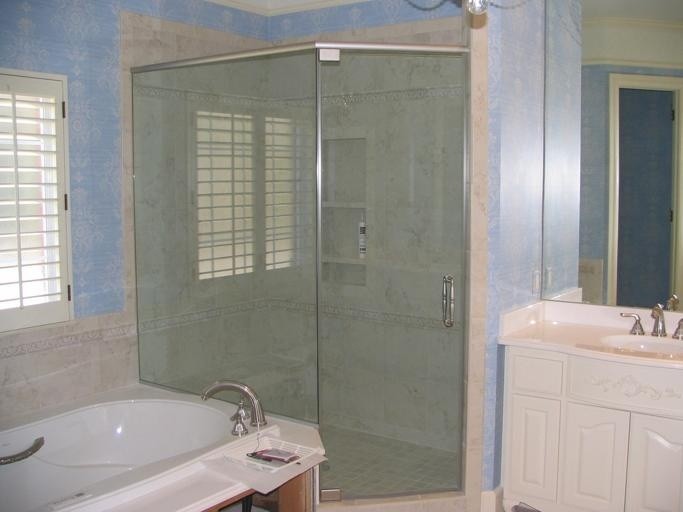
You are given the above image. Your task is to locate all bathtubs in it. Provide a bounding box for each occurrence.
[0,384,282,511]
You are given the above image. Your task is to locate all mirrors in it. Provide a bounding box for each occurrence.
[541,2,682,313]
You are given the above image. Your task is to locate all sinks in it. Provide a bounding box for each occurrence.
[601,333,683,356]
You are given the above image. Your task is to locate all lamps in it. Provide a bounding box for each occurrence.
[405,1,530,30]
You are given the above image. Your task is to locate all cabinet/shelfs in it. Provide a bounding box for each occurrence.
[496,342,681,512]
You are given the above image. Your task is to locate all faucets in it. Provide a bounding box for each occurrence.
[201,379,268,427]
[666,294,679,312]
[650,303,666,336]
